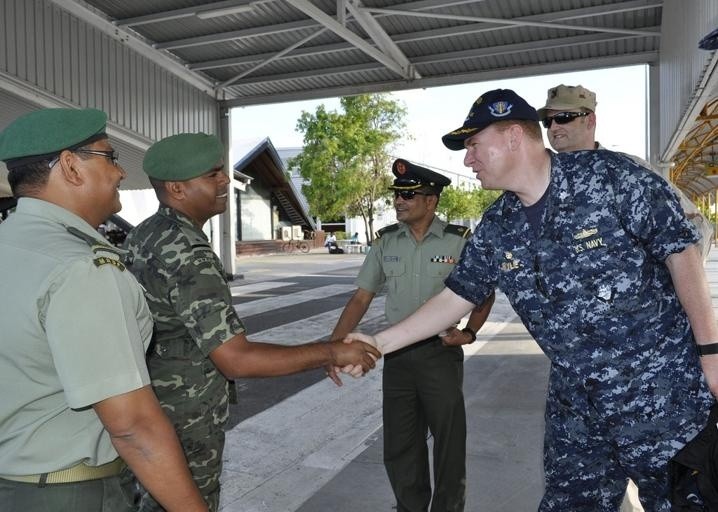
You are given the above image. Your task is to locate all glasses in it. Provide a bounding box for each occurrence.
[47,147,121,168]
[393,189,427,199]
[543,109,592,129]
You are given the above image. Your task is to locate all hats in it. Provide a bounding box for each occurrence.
[386,157,452,196]
[0,106,108,171]
[141,132,226,183]
[442,87,542,151]
[539,83,598,116]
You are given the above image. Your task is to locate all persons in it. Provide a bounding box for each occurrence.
[350,232,362,253]
[324,230,338,253]
[537,84,710,267]
[0,108,216,512]
[327,158,497,512]
[332,89,718,512]
[122,132,380,512]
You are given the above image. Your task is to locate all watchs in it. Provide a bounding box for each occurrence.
[694,343,718,355]
[464,328,478,341]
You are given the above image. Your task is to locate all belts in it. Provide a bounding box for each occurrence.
[0,457,126,485]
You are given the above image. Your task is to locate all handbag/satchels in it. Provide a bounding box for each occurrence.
[665,396,718,510]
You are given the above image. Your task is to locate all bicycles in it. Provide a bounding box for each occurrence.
[282,235,310,254]
